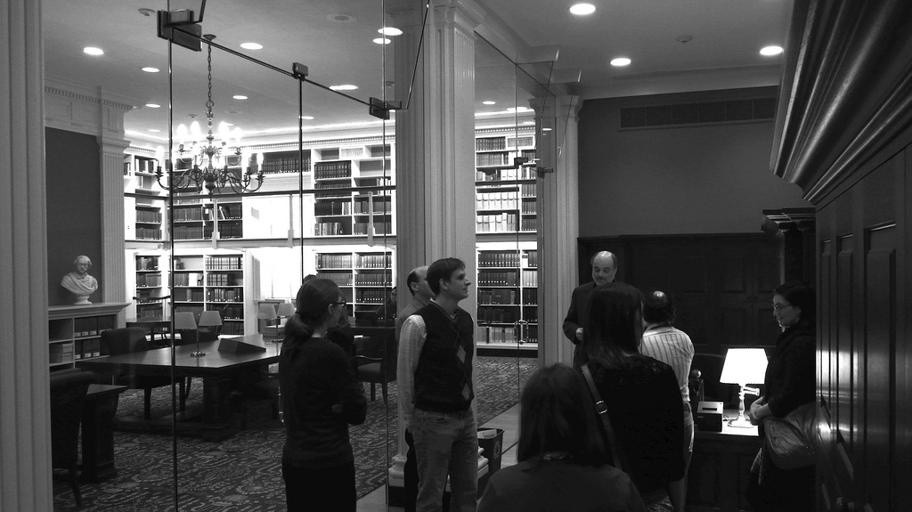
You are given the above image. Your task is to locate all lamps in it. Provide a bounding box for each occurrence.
[155,35,264,198]
[257,303,295,342]
[720,348,769,425]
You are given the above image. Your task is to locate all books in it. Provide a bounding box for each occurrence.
[206,256,243,335]
[317,254,392,304]
[477,249,536,342]
[248,151,310,175]
[125,157,242,239]
[475,136,538,232]
[312,164,392,235]
[51,319,118,367]
[169,258,205,325]
[135,256,163,320]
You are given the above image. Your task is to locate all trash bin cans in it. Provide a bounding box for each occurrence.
[477,427,504,472]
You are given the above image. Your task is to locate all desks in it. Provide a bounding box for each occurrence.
[695,407,764,507]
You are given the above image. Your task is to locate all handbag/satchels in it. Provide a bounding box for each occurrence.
[760,402,835,469]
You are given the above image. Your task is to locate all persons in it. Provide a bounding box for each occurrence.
[476,363,651,510]
[280,279,368,511]
[396,257,479,511]
[59,256,99,305]
[562,251,621,368]
[749,279,816,512]
[299,274,360,372]
[582,284,684,512]
[636,286,695,476]
[401,264,438,512]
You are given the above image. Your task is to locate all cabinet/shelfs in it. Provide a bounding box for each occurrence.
[136,249,164,324]
[578,206,817,353]
[134,148,242,243]
[475,137,537,234]
[172,255,243,335]
[314,136,391,234]
[316,253,393,329]
[478,251,537,350]
